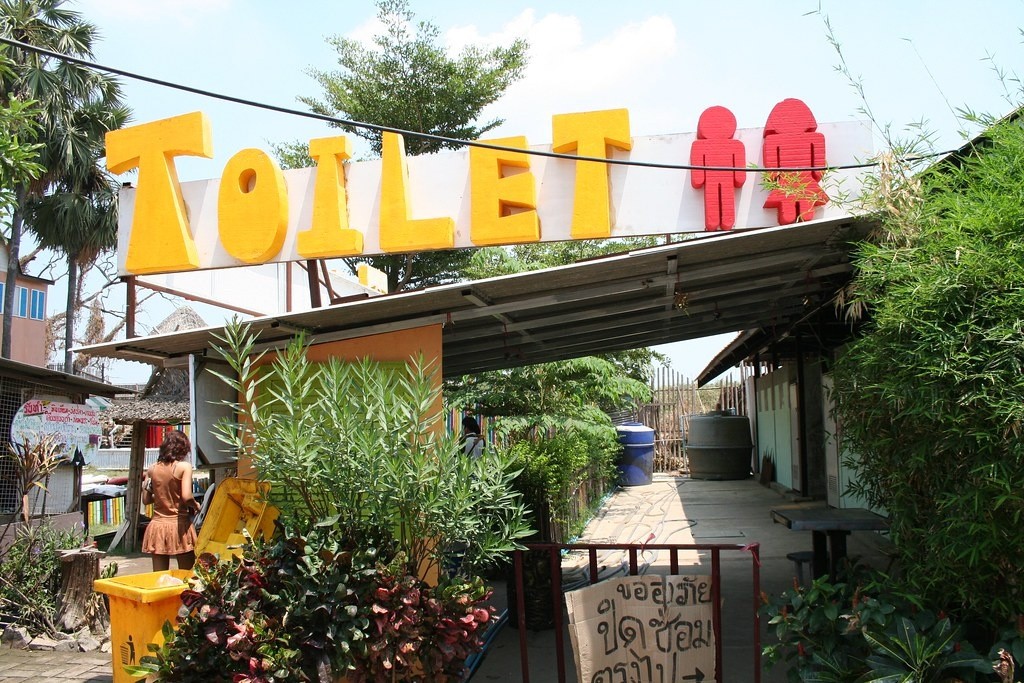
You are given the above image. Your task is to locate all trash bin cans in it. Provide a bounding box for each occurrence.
[93,481,280,683]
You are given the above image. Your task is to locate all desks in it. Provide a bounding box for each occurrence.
[773,508,890,584]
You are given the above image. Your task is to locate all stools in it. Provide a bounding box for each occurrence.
[881,553,900,581]
[786,551,862,586]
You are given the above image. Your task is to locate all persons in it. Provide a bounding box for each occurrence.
[141,429,202,572]
[458,417,486,480]
